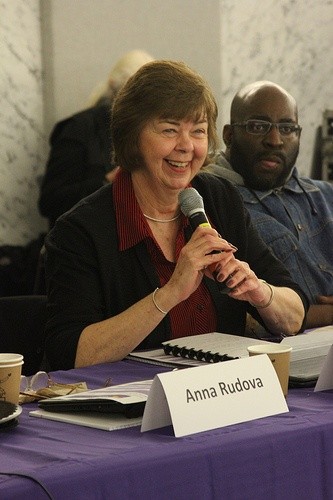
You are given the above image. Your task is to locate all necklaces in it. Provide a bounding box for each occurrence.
[142,212,182,222]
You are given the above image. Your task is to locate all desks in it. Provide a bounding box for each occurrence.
[0,359,333,500]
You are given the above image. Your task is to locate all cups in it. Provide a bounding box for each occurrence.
[247,344,292,401]
[0,353,24,405]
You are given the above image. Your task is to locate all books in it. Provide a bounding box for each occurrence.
[162,332,280,362]
[28,378,154,431]
[124,348,215,369]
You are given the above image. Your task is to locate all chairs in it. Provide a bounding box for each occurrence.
[0,296,49,376]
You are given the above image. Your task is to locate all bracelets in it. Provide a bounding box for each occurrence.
[246,279,274,310]
[152,287,167,316]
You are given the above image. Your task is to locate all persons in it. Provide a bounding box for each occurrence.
[37,60,312,377]
[200,82,332,325]
[38,48,155,230]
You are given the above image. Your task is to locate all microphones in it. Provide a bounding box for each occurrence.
[177,187,237,295]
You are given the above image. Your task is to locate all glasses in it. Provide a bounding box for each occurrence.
[230,119,302,135]
